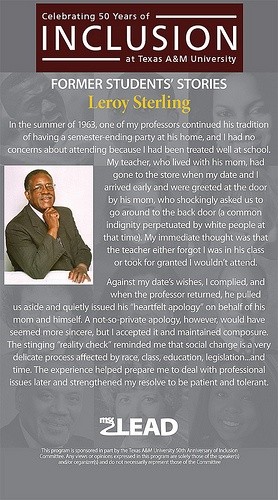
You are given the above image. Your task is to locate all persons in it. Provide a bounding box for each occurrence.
[0,72,278,449]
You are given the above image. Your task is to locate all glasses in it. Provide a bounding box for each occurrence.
[30,184,56,193]
